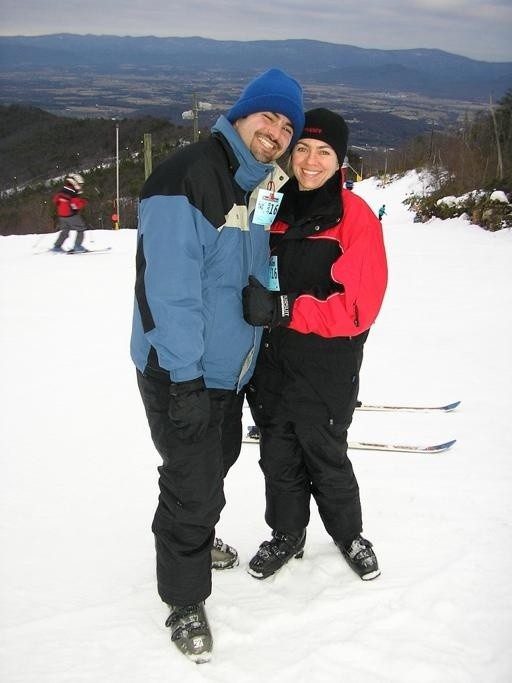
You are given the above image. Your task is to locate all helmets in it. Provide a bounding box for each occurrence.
[66,173,85,195]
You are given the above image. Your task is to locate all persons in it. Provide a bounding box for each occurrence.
[345,176,354,191]
[130,68,305,664]
[378,204,385,220]
[241,107,388,581]
[50,173,89,253]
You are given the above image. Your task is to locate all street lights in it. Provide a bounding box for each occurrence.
[111,116,127,231]
[358,155,364,180]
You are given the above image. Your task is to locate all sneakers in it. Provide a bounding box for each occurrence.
[165,530,381,664]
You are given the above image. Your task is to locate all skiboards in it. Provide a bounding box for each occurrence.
[237,400,462,455]
[33,247,112,255]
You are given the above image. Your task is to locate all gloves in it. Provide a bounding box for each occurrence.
[242,275,300,329]
[168,378,211,443]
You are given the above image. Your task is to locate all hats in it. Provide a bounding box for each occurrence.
[226,67,305,152]
[290,108,348,170]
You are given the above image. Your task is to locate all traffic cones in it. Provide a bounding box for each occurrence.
[114,222,119,230]
